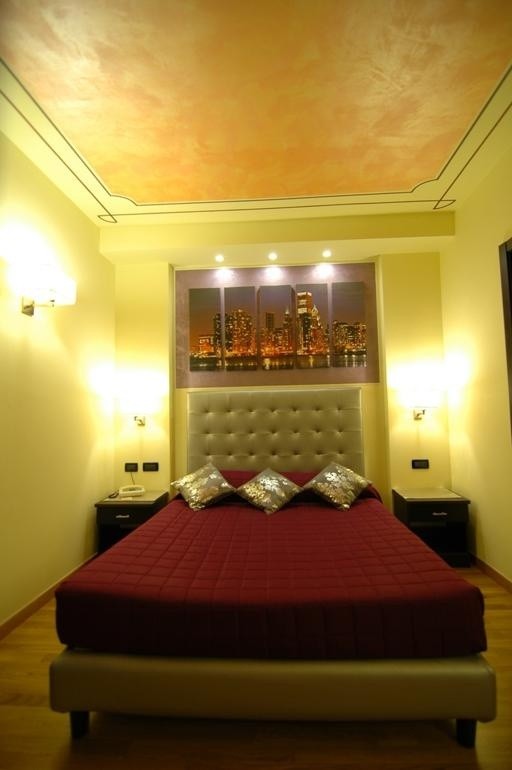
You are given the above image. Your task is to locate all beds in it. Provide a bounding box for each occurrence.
[50,387,497,749]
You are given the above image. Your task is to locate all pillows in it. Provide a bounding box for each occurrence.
[303,461,373,512]
[170,462,236,512]
[236,467,304,515]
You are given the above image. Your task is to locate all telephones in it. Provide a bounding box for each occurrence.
[119,485,145,498]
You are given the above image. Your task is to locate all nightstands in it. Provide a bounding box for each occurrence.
[93,489,168,555]
[391,488,475,569]
